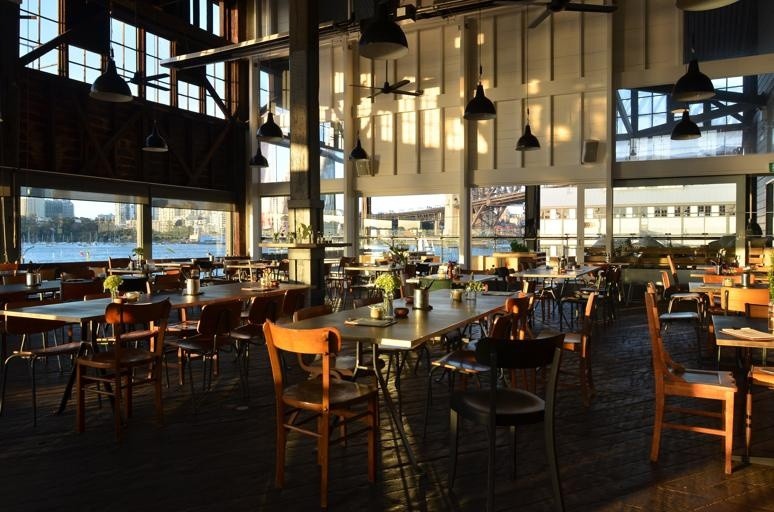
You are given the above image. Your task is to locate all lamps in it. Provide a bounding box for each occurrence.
[248,0,269,169]
[256,4,285,143]
[512,1,541,152]
[354,1,408,63]
[459,0,496,122]
[140,0,168,153]
[345,67,369,162]
[89,1,132,105]
[660,0,739,143]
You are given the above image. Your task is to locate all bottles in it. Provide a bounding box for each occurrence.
[447,261,457,279]
[316,230,333,244]
[561,255,568,274]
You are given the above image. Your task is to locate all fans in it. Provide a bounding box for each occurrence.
[93,22,174,94]
[515,0,621,32]
[347,57,423,102]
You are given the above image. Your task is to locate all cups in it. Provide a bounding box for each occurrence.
[272,281,278,286]
[716,265,723,275]
[369,306,384,319]
[272,260,278,266]
[724,278,733,287]
[185,276,201,295]
[130,259,134,270]
[439,271,445,279]
[26,272,43,285]
[209,255,214,262]
[742,273,750,286]
[144,264,148,270]
[113,299,124,304]
[450,289,463,300]
[260,277,266,285]
[191,258,197,264]
[413,288,430,308]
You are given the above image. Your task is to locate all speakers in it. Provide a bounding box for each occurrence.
[356,159,373,176]
[580,139,598,163]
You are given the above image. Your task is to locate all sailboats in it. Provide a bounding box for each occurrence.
[412,237,436,256]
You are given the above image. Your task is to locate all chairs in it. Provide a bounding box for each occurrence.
[262,288,599,509]
[646,236,773,476]
[1,250,441,435]
[406,253,627,332]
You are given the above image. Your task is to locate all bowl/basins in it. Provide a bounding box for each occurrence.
[406,296,414,303]
[394,308,409,317]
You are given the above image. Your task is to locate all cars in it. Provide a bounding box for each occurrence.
[583,232,692,269]
[715,233,774,272]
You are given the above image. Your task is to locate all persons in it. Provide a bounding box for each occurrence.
[747,218,762,238]
